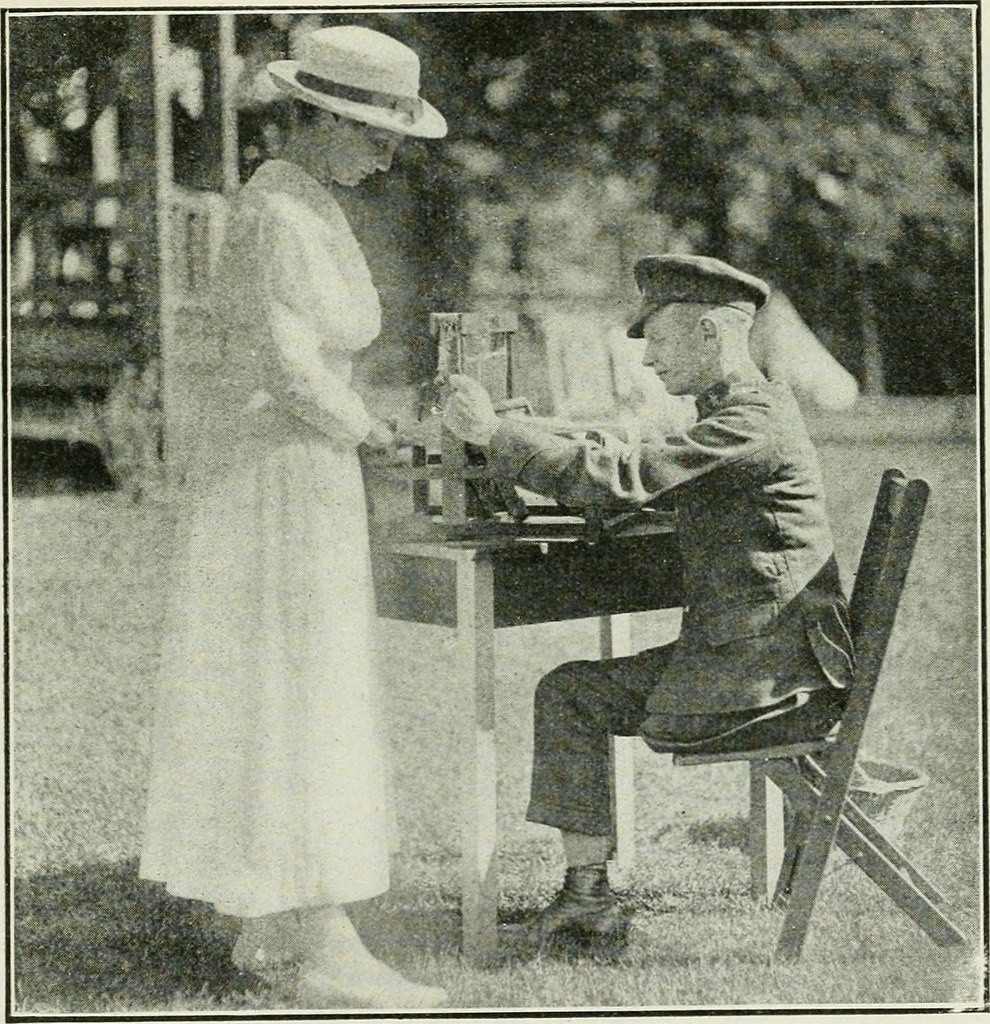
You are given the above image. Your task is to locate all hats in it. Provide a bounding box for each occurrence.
[626,252,770,339]
[265,26,449,140]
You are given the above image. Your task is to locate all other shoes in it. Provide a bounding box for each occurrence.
[496,866,630,965]
[292,951,448,1013]
[232,934,309,994]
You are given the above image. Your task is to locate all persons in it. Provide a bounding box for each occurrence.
[139,27,449,1010]
[442,254,854,950]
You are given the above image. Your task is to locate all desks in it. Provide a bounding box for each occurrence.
[367,519,787,967]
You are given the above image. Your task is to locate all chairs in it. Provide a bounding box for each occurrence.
[672,465,967,966]
